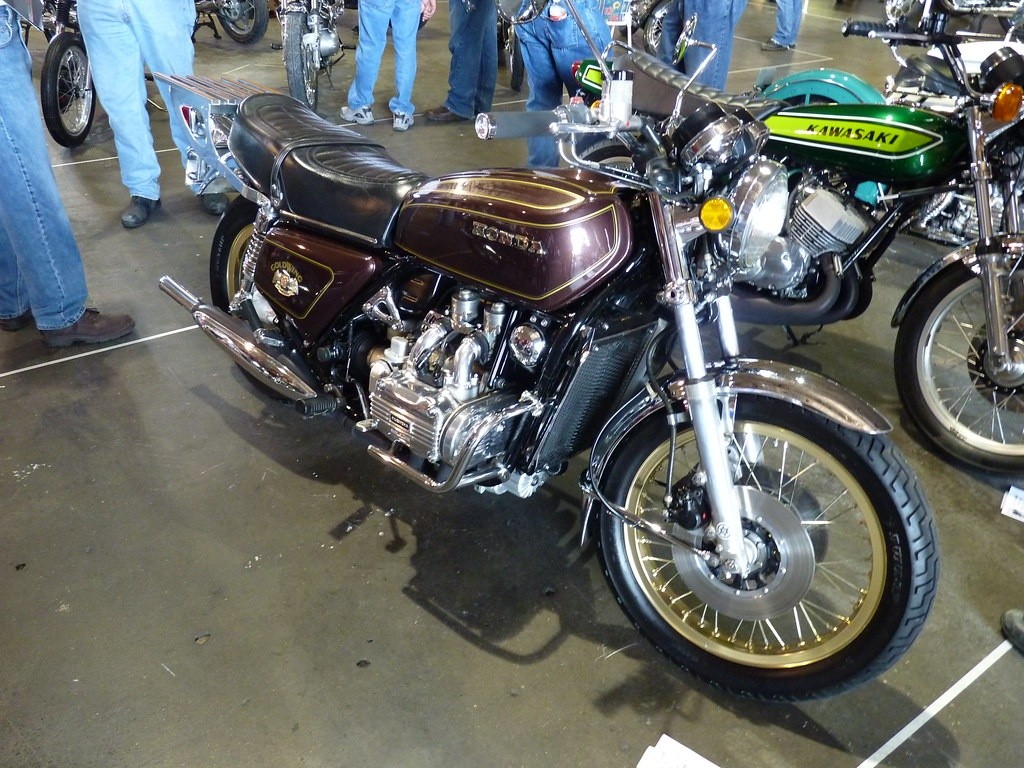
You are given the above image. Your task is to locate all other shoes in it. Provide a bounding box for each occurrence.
[760,39,795,50]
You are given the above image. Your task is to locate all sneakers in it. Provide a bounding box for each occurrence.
[422,105,467,122]
[393,110,414,130]
[340,105,375,125]
[0,308,35,332]
[120,196,161,228]
[40,307,135,348]
[201,192,229,216]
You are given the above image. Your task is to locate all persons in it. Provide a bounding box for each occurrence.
[657,0,748,92]
[0,1,136,346]
[339,0,437,132]
[761,0,802,51]
[426,0,499,124]
[77,0,230,226]
[497,0,615,167]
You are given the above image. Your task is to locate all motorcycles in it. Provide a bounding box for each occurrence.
[12,0,1024,703]
